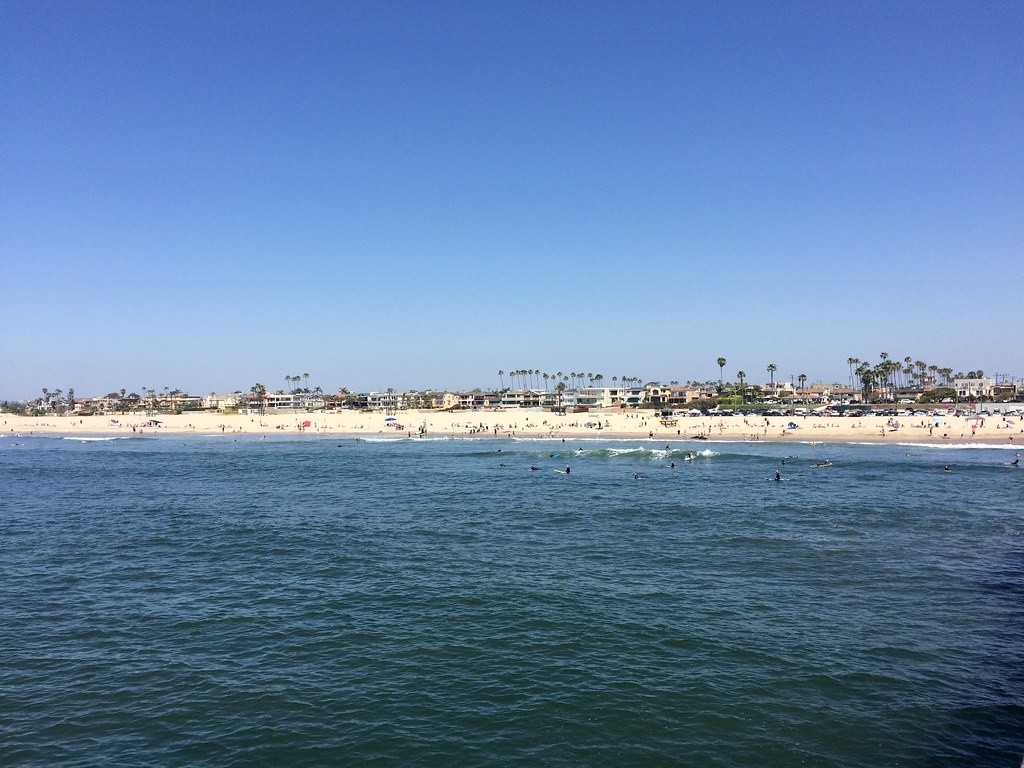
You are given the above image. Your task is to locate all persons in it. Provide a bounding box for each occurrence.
[566,465,571,475]
[0,411,1024,480]
